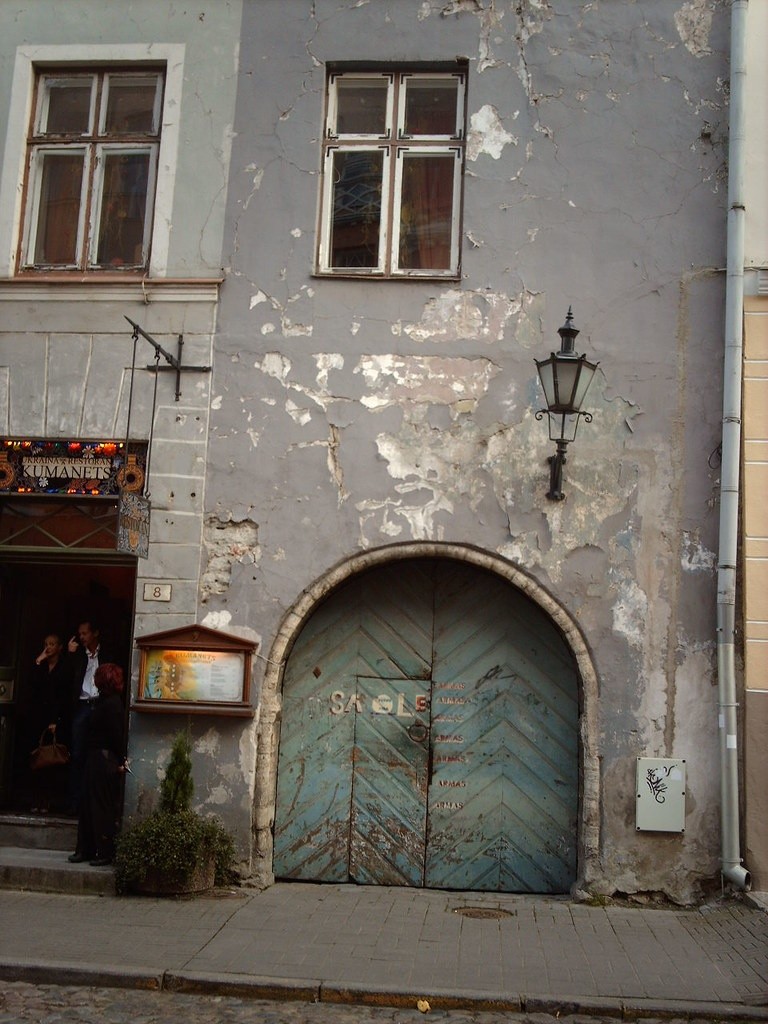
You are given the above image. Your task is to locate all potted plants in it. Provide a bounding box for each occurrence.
[110,730,238,898]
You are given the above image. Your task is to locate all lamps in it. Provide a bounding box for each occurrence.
[533,306,602,501]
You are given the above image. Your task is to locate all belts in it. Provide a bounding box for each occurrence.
[76,699,97,704]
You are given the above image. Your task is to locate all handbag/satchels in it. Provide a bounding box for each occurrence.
[29,728,71,770]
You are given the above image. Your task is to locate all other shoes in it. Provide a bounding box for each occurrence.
[40,799,50,813]
[30,798,40,813]
[68,847,92,862]
[64,808,79,816]
[89,857,113,866]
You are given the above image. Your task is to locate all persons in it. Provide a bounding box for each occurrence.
[28,621,128,866]
